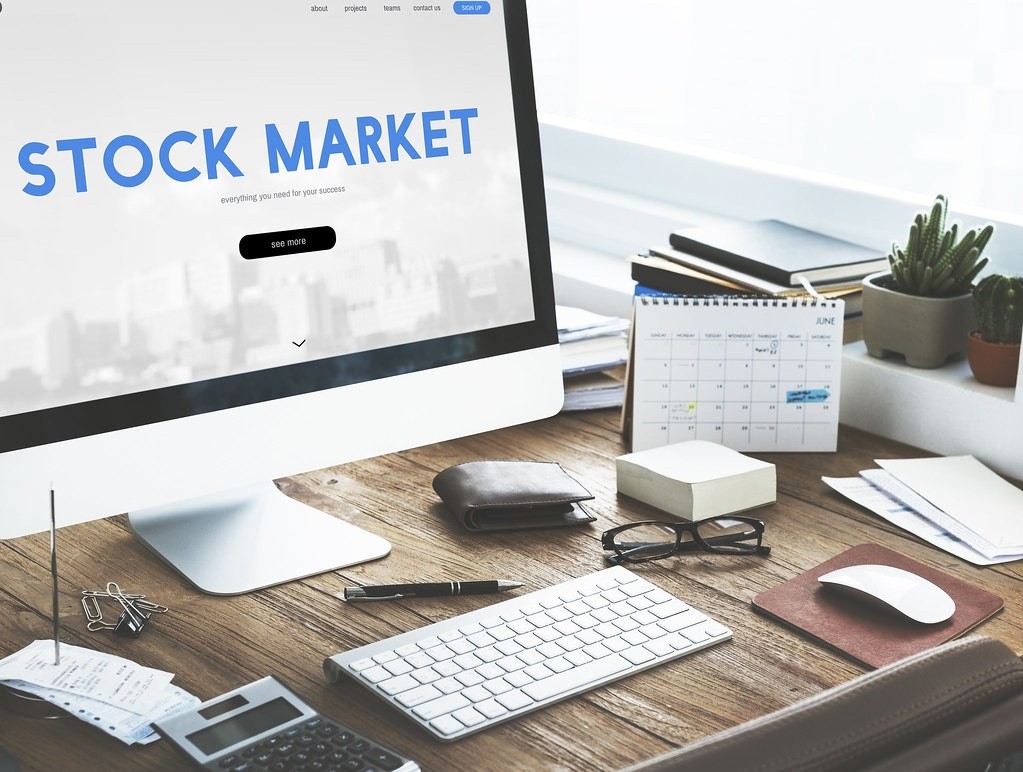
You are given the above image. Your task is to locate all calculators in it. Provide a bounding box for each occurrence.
[150,674,424,772]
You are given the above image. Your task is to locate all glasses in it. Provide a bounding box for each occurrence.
[601,515,771,567]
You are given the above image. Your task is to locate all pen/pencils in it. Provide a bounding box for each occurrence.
[344,579,524,603]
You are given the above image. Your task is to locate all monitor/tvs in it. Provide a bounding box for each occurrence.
[0,0,566,593]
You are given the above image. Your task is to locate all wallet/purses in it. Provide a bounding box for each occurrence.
[431,460,597,533]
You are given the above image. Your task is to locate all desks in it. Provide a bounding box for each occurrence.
[0,367,1023,772]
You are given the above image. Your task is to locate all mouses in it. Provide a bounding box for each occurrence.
[817,564,956,626]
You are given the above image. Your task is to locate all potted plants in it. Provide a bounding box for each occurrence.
[861,192,993,369]
[967,274,1023,389]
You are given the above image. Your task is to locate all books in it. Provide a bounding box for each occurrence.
[631,218,892,313]
[556,306,629,412]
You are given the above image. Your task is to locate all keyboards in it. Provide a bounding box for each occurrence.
[325,567,735,744]
